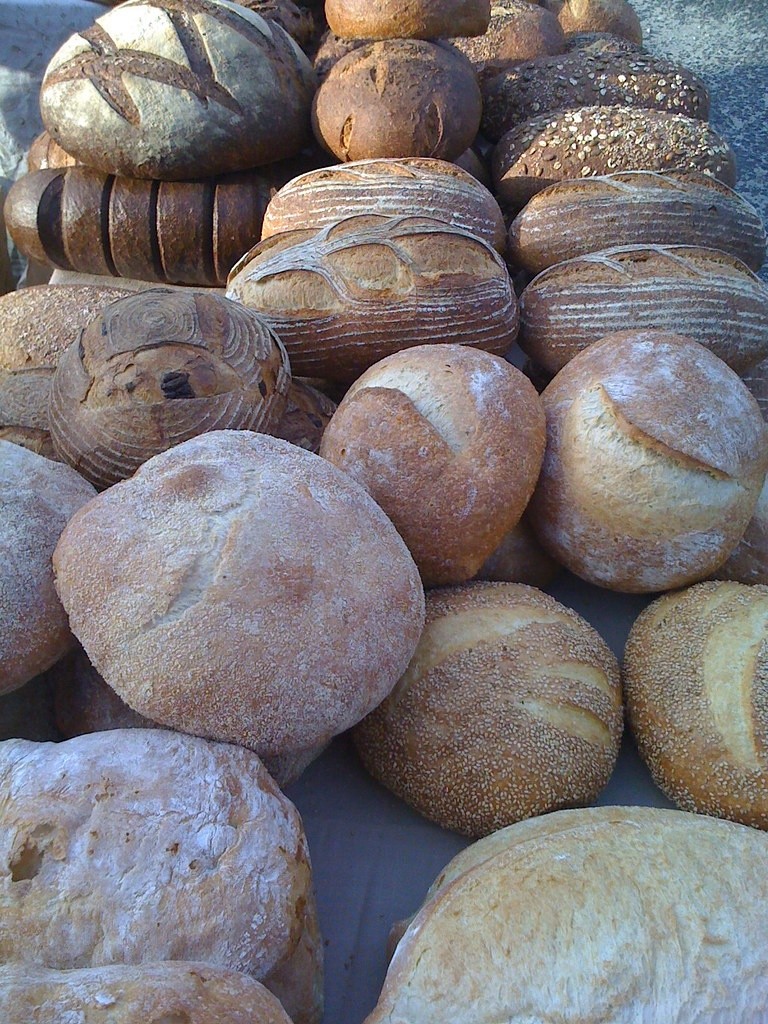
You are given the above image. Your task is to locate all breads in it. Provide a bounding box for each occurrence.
[0,0,768,1024]
